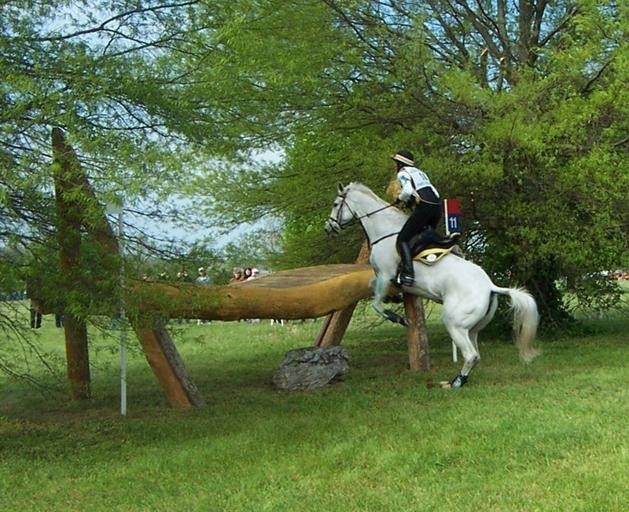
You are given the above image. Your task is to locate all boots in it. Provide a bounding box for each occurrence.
[390,241,414,286]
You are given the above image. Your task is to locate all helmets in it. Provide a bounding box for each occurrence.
[390,150,415,167]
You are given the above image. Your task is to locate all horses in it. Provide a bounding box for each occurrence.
[323,180,541,389]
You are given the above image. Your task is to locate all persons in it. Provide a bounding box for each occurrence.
[391,150,442,287]
[142,267,285,327]
[26,284,41,329]
[56,314,63,326]
